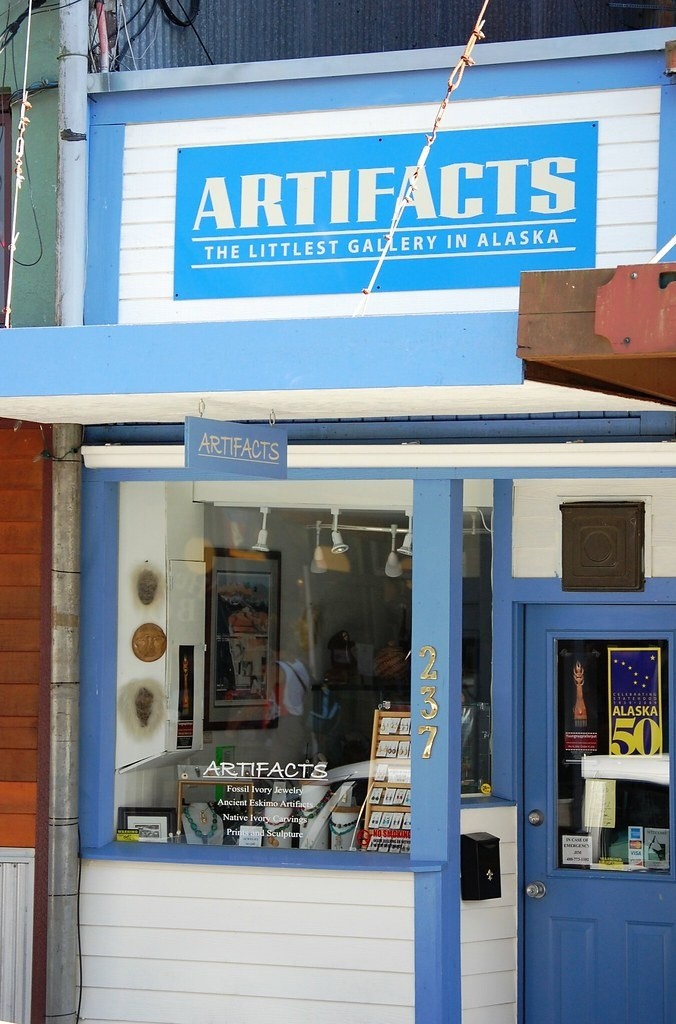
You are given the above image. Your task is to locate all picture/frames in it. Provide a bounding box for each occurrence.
[204,547,282,732]
[118,807,177,844]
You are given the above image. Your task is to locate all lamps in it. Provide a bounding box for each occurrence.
[251,507,272,552]
[310,520,328,574]
[396,508,412,557]
[329,508,349,554]
[385,524,404,578]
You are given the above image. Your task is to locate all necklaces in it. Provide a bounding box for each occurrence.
[190,806,209,824]
[184,805,218,845]
[263,790,357,849]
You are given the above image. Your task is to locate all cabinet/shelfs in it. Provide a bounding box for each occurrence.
[362,709,411,851]
